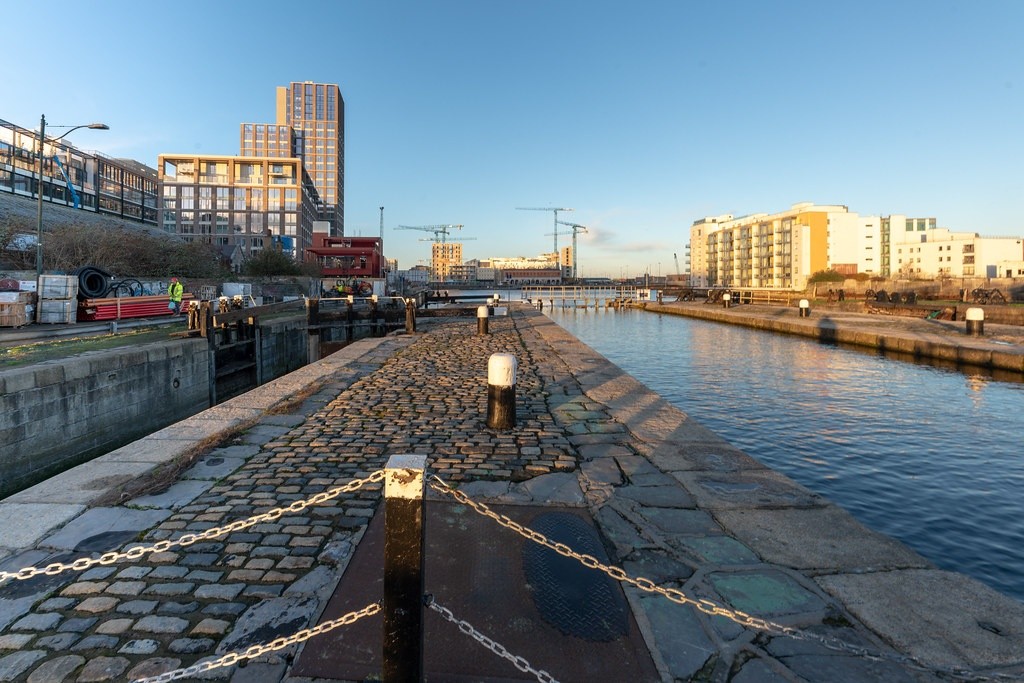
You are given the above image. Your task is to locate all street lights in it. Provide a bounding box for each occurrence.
[36,114,111,295]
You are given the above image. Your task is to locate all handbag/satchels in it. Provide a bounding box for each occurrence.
[168,296,175,309]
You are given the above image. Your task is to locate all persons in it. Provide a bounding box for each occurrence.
[338,284,344,293]
[167,277,183,318]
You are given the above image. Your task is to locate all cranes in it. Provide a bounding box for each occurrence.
[392,223,478,244]
[515,207,589,283]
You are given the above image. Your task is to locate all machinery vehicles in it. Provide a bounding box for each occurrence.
[323,274,373,298]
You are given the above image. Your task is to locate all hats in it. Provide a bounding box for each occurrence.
[171,278,178,282]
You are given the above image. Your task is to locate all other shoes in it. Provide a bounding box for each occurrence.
[172,314,180,318]
